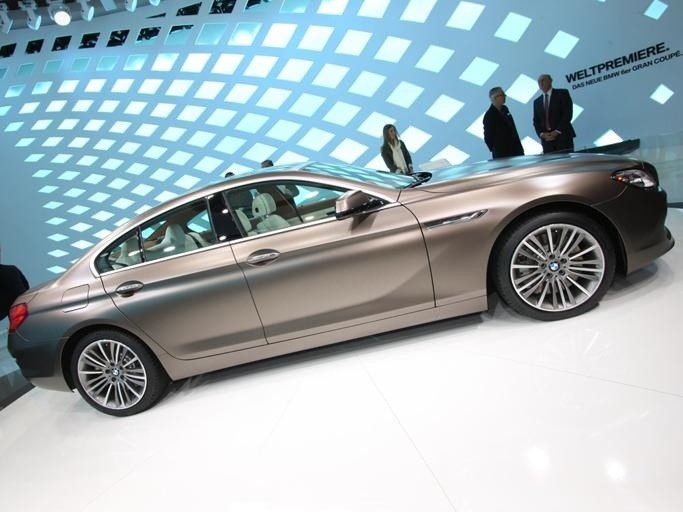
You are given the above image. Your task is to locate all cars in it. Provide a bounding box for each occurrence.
[7,153,675,416]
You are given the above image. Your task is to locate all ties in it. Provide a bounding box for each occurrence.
[545,93,550,131]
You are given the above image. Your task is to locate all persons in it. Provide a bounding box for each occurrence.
[224,172,253,220]
[533,73,577,156]
[259,160,299,207]
[379,123,412,176]
[0,264,29,323]
[483,88,525,160]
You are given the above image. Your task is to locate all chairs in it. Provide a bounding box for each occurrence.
[235,191,291,237]
[111,225,210,275]
